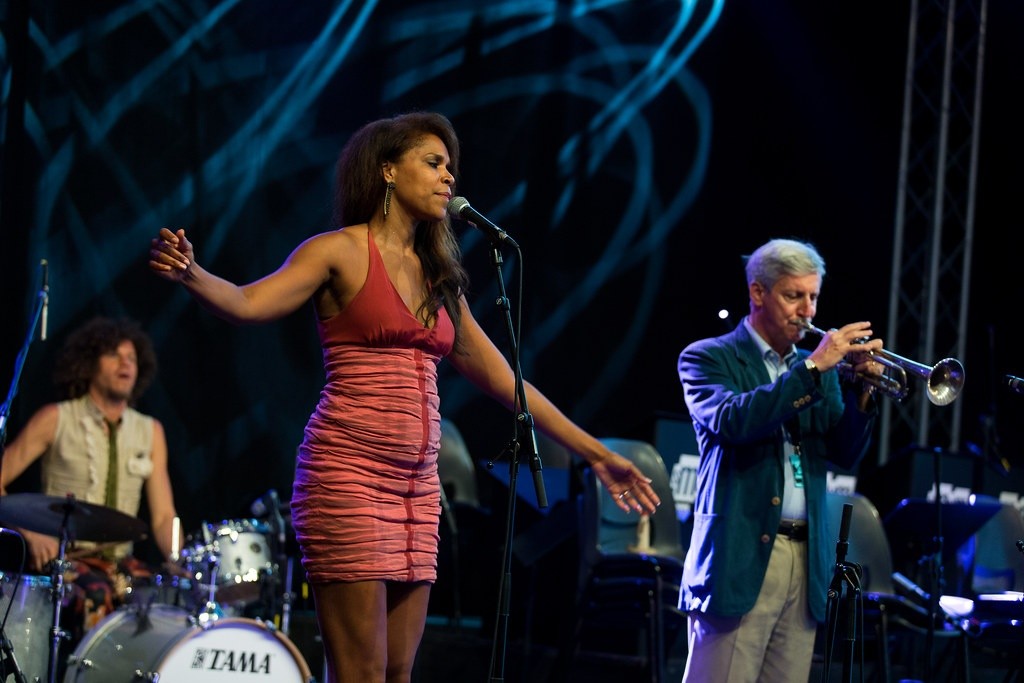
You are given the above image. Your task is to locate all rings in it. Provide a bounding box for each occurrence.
[872,361,875,366]
[621,491,629,496]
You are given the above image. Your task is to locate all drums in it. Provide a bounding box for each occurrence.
[0,571,65,683]
[62,520,318,683]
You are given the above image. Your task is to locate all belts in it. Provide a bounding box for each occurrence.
[777,522,808,542]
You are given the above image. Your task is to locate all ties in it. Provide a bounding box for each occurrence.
[100,416,122,560]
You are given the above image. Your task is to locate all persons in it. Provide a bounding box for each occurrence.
[0,315,185,571]
[150,112,662,683]
[678,240,883,683]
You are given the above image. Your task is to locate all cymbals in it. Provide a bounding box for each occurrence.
[0,492,146,543]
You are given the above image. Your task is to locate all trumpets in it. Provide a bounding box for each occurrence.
[801,316,965,407]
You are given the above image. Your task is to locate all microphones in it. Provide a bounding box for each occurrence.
[1005,375,1024,391]
[40,263,49,341]
[447,196,520,250]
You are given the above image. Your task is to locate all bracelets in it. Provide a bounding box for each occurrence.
[805,358,821,387]
